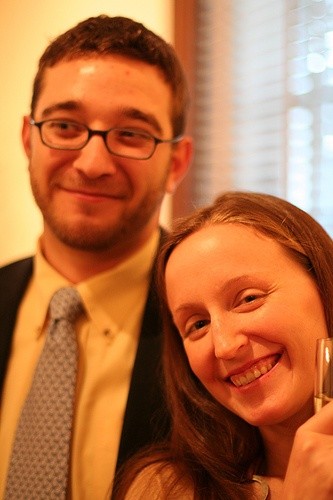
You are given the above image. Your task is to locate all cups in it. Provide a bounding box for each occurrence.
[314,334,333,421]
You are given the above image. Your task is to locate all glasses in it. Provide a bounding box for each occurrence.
[28,118,182,159]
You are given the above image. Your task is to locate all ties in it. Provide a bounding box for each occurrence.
[4,284,85,499]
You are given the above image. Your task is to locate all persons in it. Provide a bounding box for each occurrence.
[115,192,332,499]
[0,14,193,500]
